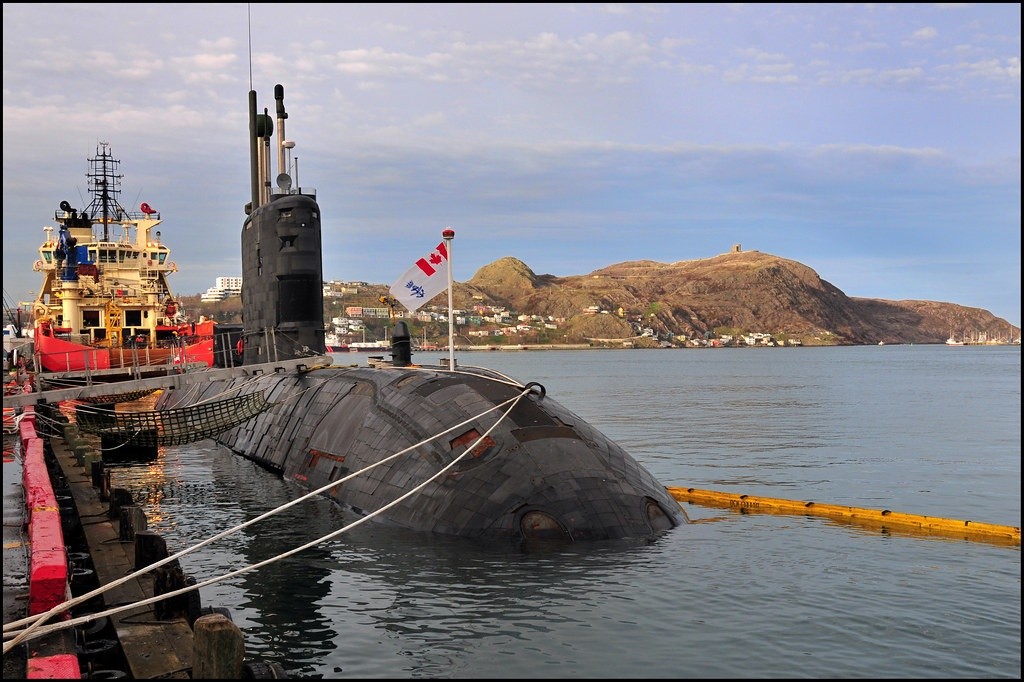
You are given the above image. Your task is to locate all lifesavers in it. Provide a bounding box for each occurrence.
[60,201,70,210]
[141,202,149,212]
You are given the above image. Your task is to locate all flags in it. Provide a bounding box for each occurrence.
[389,241,448,313]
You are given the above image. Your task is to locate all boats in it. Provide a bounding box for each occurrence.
[3,325,18,370]
[878,341,885,346]
[945,339,967,345]
[20,138,215,372]
